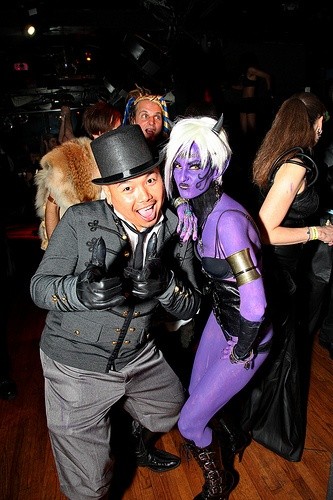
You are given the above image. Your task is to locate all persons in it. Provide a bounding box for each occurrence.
[237,95,333,463]
[30,63,333,275]
[29,124,203,494]
[161,115,274,495]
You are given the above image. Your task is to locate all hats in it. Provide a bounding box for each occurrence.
[91,124,163,185]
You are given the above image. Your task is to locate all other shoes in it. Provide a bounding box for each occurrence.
[222,429,252,469]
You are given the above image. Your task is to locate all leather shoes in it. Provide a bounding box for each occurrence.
[0,381,17,400]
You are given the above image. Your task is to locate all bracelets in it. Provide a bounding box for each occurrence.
[172,199,189,208]
[306,226,318,241]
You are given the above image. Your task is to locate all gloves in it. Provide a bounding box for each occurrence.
[123,232,171,300]
[76,236,128,310]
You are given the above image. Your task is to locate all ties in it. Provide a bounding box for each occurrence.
[120,219,155,272]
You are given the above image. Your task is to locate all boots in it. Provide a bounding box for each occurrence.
[113,415,180,472]
[183,430,234,500]
